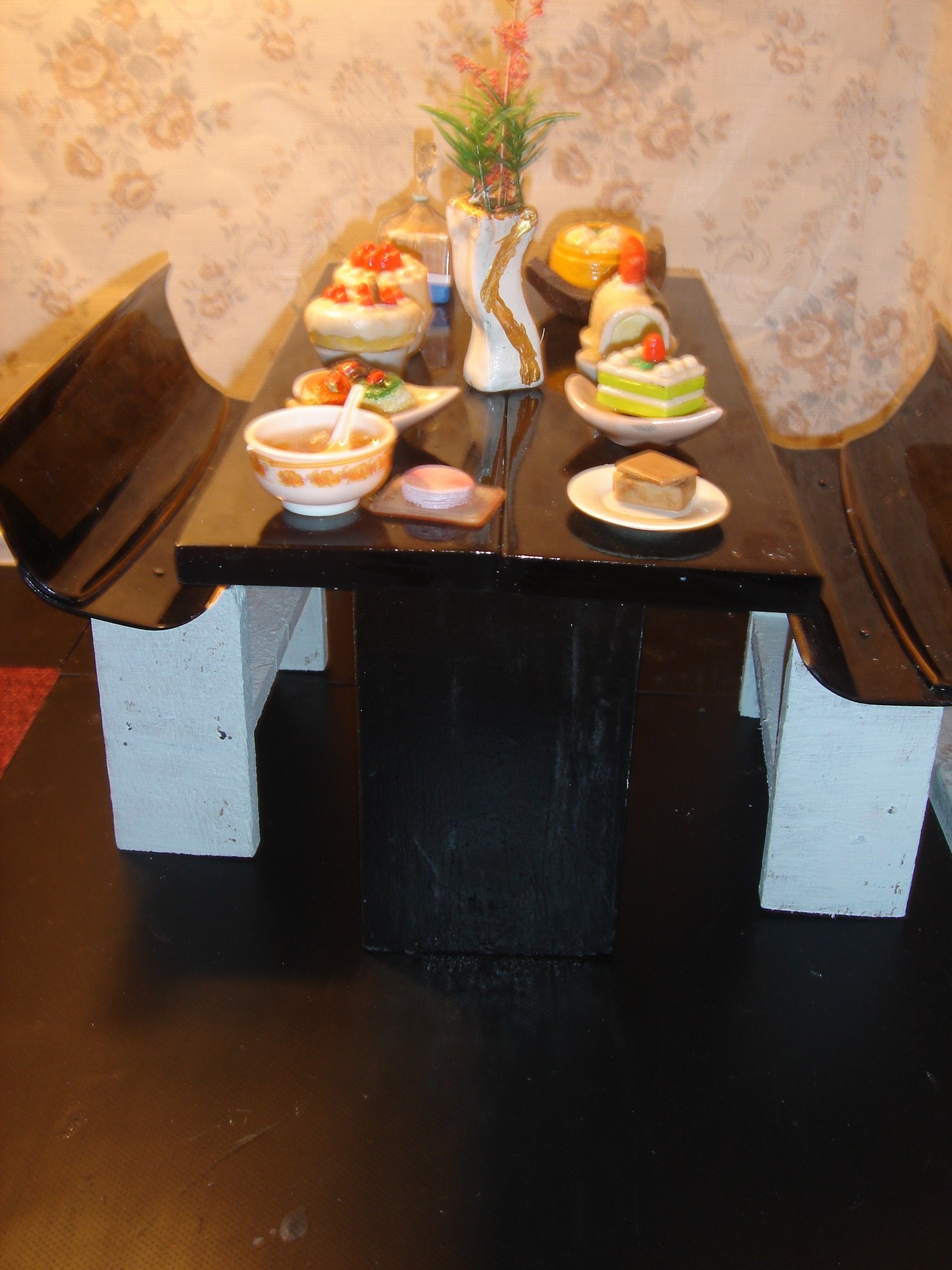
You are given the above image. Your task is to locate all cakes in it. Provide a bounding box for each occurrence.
[329,234,433,324]
[596,328,708,421]
[546,209,650,291]
[578,236,669,346]
[608,449,701,515]
[301,271,425,353]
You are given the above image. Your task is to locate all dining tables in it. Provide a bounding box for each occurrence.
[167,270,818,918]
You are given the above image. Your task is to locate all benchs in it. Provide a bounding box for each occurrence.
[733,313,952,921]
[0,258,334,863]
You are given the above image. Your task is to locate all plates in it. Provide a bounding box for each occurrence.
[563,376,726,448]
[567,462,730,532]
[527,259,593,322]
[370,473,507,527]
[286,369,464,439]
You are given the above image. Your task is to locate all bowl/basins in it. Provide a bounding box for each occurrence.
[243,404,395,517]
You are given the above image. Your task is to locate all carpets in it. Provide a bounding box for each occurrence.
[0,667,60,783]
